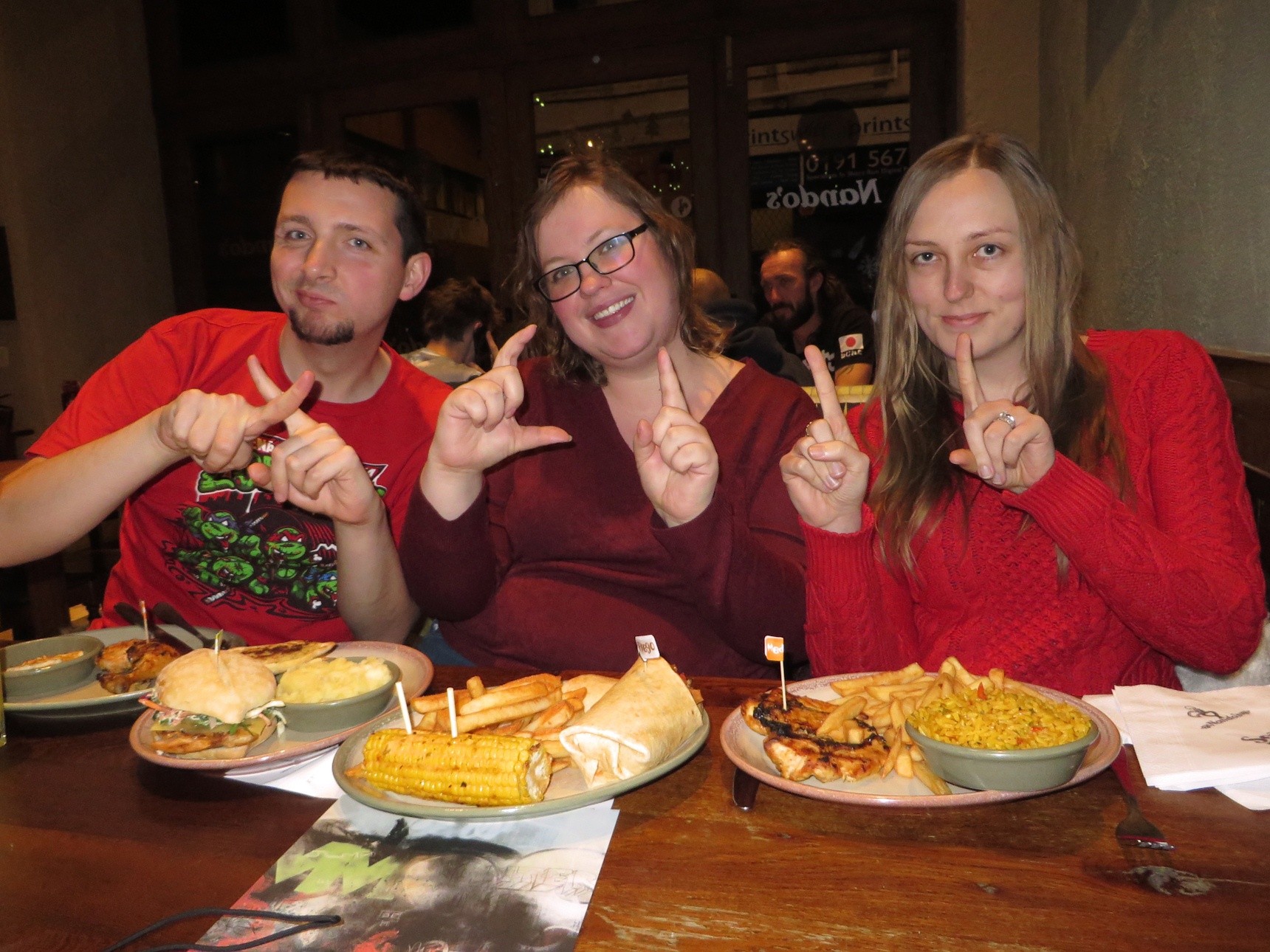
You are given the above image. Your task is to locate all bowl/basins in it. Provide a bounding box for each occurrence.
[270,656,400,732]
[905,718,1099,792]
[0,635,103,697]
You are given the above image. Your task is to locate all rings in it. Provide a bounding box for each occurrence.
[1000,410,1017,431]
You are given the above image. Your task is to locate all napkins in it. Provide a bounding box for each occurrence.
[1080,683,1270,811]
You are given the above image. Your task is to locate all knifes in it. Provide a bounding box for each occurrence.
[732,762,760,810]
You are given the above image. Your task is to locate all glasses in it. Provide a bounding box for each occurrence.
[533,219,652,303]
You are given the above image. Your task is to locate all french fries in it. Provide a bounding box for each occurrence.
[816,658,1051,794]
[412,673,587,772]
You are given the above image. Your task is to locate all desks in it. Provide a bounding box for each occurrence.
[1,660,1270,952]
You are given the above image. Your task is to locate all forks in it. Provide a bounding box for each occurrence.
[1111,744,1174,850]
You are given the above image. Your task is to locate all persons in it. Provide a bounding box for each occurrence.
[750,239,877,387]
[397,144,824,681]
[399,274,500,393]
[0,140,456,644]
[779,129,1268,700]
[683,266,815,386]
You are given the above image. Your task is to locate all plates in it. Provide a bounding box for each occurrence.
[719,671,1121,808]
[3,624,247,722]
[330,703,710,822]
[128,640,434,776]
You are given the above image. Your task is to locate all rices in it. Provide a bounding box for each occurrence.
[907,681,1092,751]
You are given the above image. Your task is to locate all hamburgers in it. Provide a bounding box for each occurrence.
[138,648,286,760]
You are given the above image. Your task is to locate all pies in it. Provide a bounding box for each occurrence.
[741,689,890,781]
[232,640,336,675]
[562,656,702,785]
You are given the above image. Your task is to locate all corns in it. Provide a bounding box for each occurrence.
[361,729,553,803]
